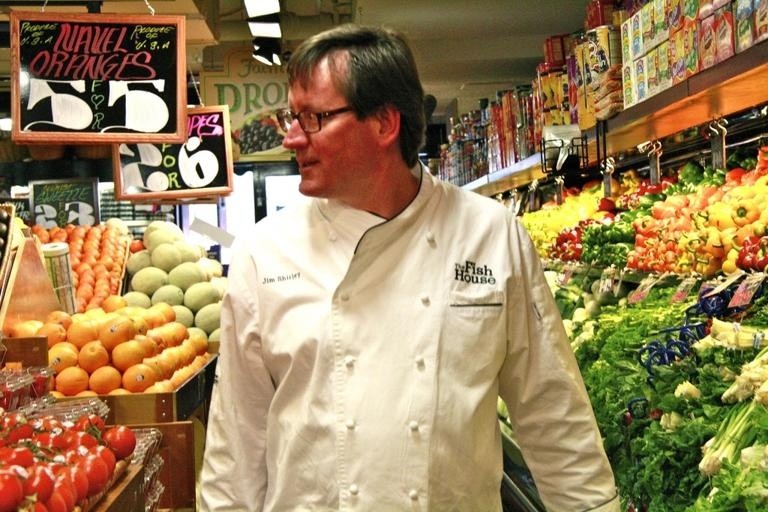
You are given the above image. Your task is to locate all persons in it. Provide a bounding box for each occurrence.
[193,25,621,512]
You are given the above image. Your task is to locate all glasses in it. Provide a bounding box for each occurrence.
[276,106,350,133]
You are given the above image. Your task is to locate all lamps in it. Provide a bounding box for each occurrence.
[243,0,283,18]
[252,36,284,67]
[248,15,284,39]
